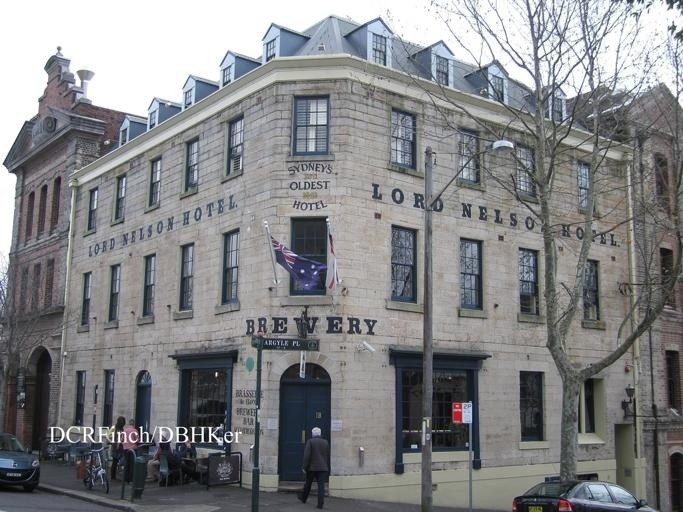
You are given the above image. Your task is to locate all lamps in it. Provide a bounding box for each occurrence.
[621,383,635,418]
[296,313,308,339]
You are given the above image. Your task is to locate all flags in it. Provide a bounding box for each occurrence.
[327,223,344,289]
[270,233,327,290]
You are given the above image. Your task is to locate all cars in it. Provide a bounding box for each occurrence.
[511,479,658,510]
[0,433,40,492]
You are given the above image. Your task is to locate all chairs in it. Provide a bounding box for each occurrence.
[48,438,221,487]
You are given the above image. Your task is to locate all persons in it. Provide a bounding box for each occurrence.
[180,435,199,482]
[145,439,173,482]
[297,427,330,509]
[122,419,140,482]
[111,416,125,482]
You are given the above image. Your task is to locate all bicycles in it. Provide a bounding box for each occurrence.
[74,446,109,492]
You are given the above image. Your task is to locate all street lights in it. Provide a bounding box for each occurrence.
[416,138,514,511]
